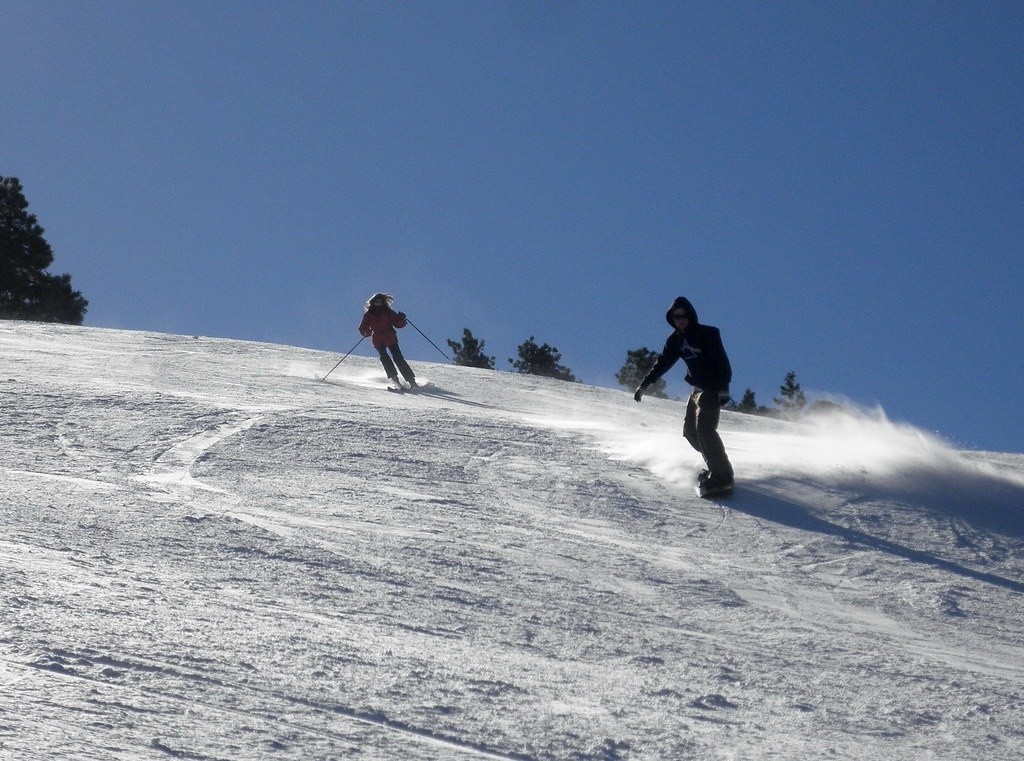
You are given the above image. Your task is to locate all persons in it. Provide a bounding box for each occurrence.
[635,296,734,487]
[358,294,418,390]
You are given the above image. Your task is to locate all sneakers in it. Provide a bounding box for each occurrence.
[390,380,418,392]
[697,469,736,498]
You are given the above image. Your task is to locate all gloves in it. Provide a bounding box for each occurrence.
[362,330,374,336]
[720,393,731,406]
[398,311,407,321]
[634,387,643,402]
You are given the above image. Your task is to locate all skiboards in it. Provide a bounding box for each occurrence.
[386,385,424,395]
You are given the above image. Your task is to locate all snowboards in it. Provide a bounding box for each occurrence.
[698,471,736,498]
